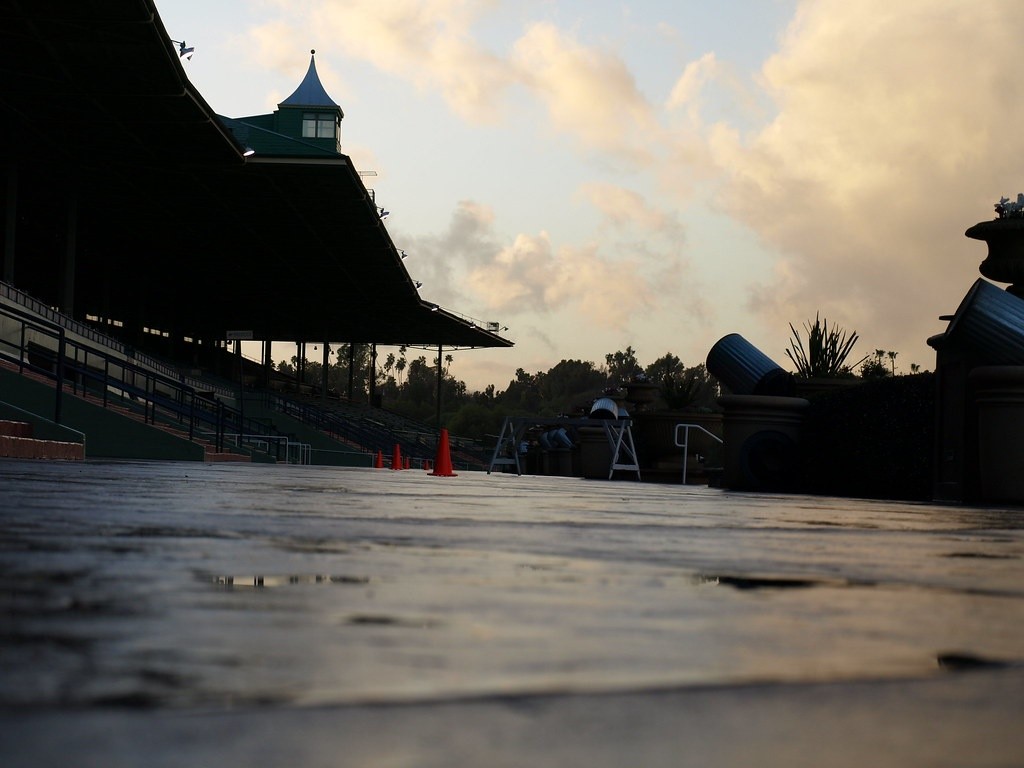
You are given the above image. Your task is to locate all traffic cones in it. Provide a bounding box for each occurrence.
[427,428,459,477]
[387,444,404,471]
[404,456,411,470]
[424,459,430,470]
[375,449,384,468]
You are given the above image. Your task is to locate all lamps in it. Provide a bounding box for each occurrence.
[401,252,407,259]
[379,212,389,220]
[180,48,194,60]
[431,305,438,312]
[504,327,508,331]
[470,322,476,328]
[416,283,422,288]
[243,148,255,157]
